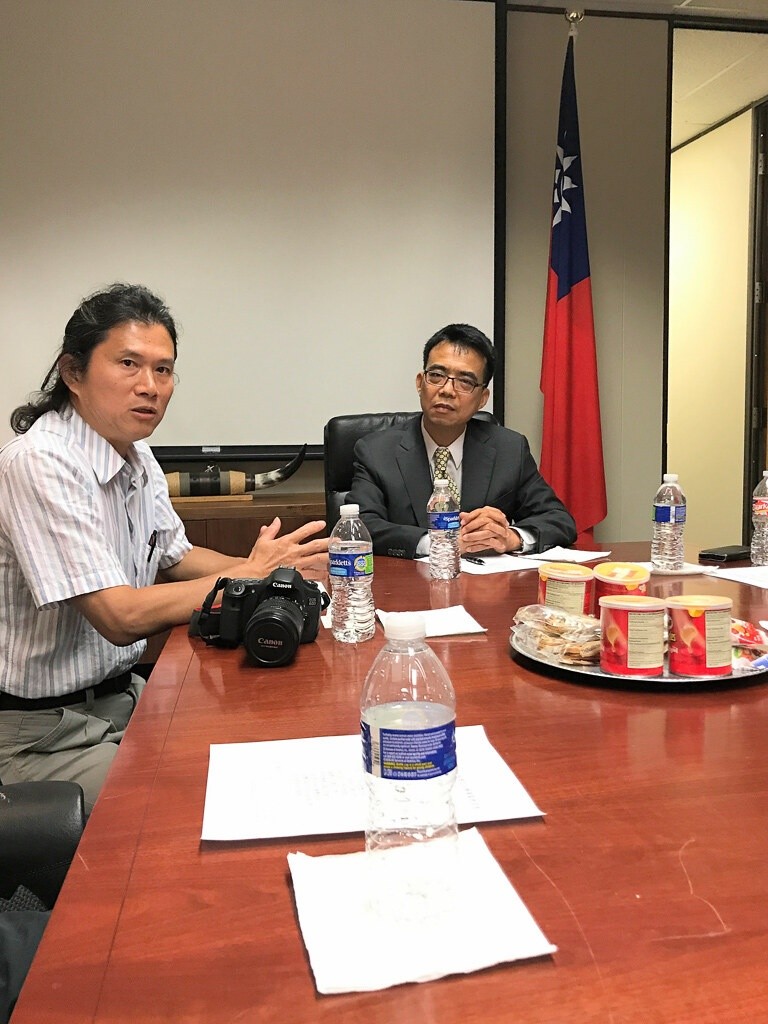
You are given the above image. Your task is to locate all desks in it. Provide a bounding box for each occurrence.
[7,541,768,1024]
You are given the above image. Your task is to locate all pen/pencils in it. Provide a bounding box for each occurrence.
[464,556,486,565]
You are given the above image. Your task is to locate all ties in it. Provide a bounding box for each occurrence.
[433,448,461,512]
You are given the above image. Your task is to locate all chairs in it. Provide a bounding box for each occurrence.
[324,410,496,538]
[0,779,83,908]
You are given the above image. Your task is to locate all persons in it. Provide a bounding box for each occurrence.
[345,323,578,560]
[0,281,342,818]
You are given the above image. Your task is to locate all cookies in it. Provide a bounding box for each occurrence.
[531,614,599,665]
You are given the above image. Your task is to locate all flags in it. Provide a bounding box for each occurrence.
[539,34,608,542]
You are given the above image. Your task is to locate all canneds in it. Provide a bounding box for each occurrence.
[598,595,666,677]
[537,562,594,615]
[594,562,650,619]
[663,595,733,679]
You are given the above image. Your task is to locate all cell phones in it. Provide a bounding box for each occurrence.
[698,546,750,560]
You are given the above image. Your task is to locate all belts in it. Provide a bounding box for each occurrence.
[0,668,132,711]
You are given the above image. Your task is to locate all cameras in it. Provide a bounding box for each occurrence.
[218,567,322,665]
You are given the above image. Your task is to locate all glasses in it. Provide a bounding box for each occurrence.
[423,370,487,394]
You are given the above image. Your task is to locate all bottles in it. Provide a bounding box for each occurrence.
[750,470,768,566]
[651,474,685,570]
[329,504,375,644]
[360,613,459,945]
[427,479,462,581]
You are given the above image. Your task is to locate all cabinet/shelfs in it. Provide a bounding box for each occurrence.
[131,494,328,664]
